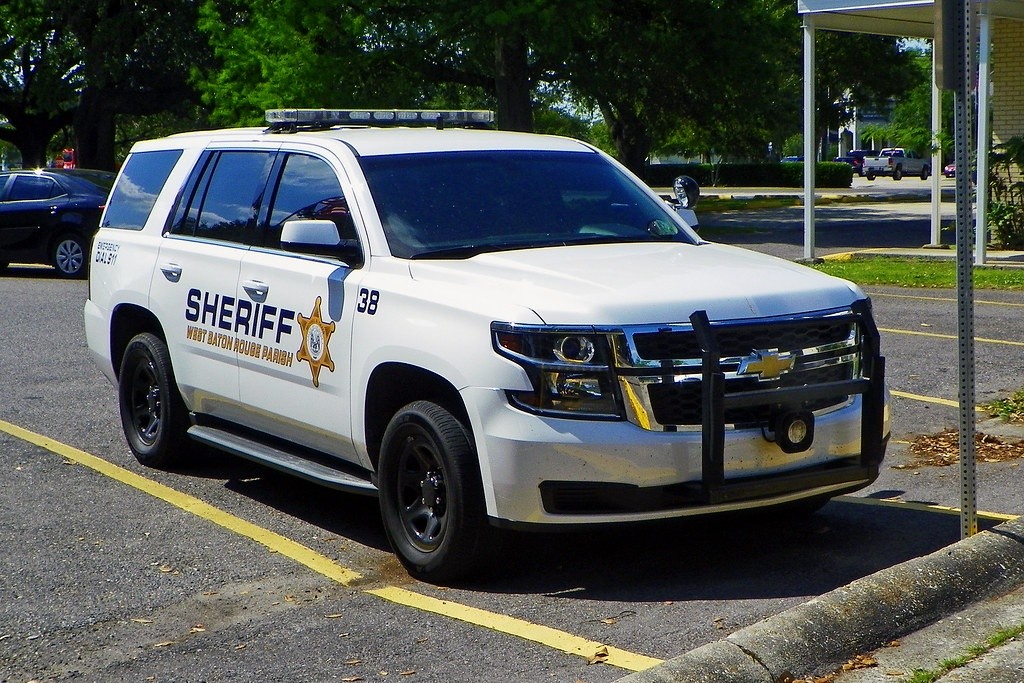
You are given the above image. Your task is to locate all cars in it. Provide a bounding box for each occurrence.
[0,167,119,280]
[944,161,955,178]
[779,155,804,162]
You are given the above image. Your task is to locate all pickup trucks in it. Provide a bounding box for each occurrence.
[833,149,881,177]
[862,147,932,181]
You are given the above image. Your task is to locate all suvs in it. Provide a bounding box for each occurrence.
[82,106,890,580]
